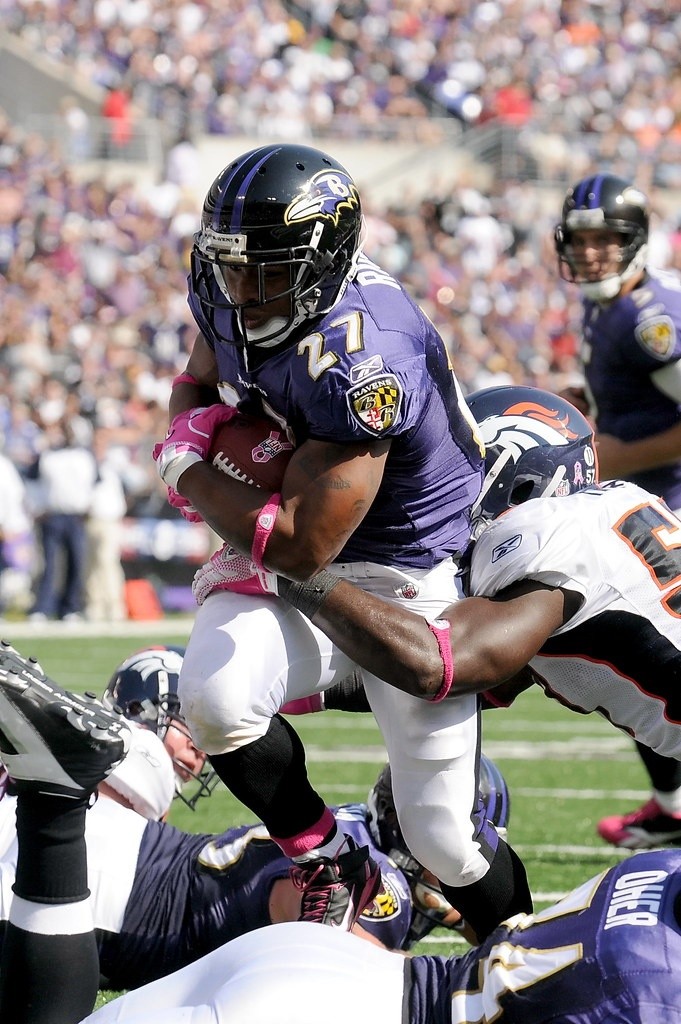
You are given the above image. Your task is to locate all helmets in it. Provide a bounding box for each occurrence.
[100,644,225,812]
[551,176,648,302]
[187,143,362,377]
[363,752,512,931]
[452,383,601,568]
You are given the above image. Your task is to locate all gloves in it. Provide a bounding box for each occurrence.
[164,484,204,526]
[191,540,286,605]
[150,405,236,490]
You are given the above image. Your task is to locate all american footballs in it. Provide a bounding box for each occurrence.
[207,413,295,496]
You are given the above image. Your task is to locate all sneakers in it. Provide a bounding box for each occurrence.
[290,833,381,934]
[0,640,134,804]
[597,796,680,847]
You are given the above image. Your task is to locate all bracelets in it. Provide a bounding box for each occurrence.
[423,619,453,702]
[250,493,281,574]
[172,375,199,387]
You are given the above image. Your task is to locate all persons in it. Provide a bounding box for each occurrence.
[0,144,680,1024]
[0,0,680,628]
